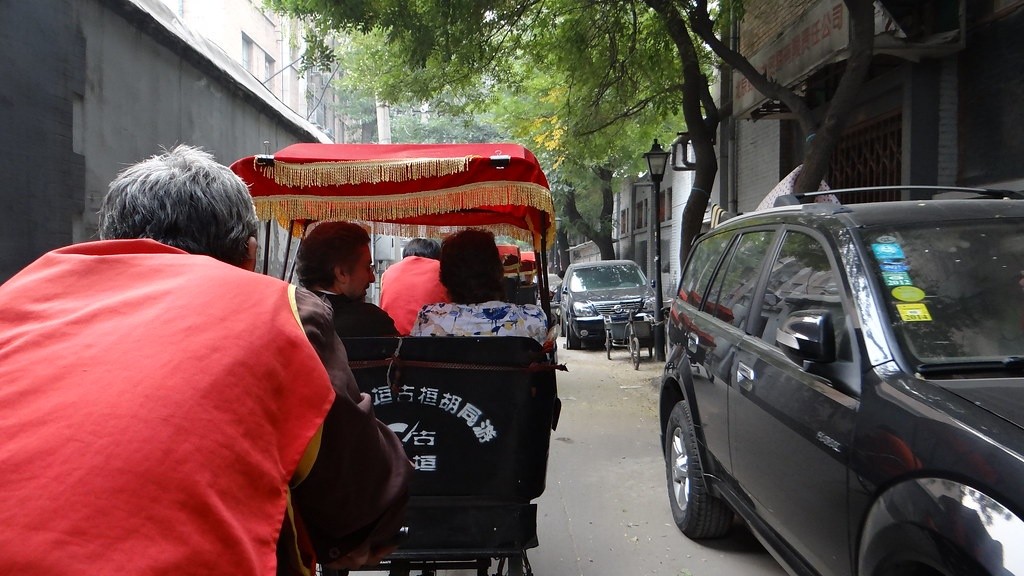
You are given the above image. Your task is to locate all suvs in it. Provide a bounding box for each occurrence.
[654,182,1024,576]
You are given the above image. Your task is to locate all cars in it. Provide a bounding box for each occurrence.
[551,259,656,351]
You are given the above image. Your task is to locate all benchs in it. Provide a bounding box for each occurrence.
[322,335,561,557]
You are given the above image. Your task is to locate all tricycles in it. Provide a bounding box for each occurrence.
[229,142,567,576]
[602,298,654,371]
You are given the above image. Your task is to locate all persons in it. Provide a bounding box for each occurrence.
[296,221,550,359]
[0,145,412,576]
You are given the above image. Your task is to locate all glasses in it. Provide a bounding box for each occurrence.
[352,263,374,273]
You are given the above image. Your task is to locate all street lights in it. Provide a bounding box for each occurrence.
[641,138,672,362]
[554,215,562,273]
